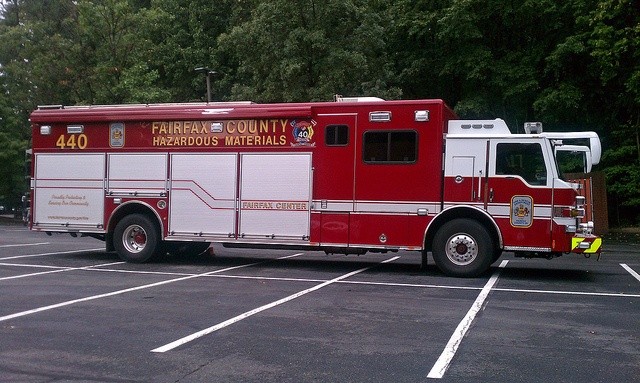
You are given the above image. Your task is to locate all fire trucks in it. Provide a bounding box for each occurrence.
[26,93,603,278]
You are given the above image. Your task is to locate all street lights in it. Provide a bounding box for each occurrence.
[195,66,219,103]
